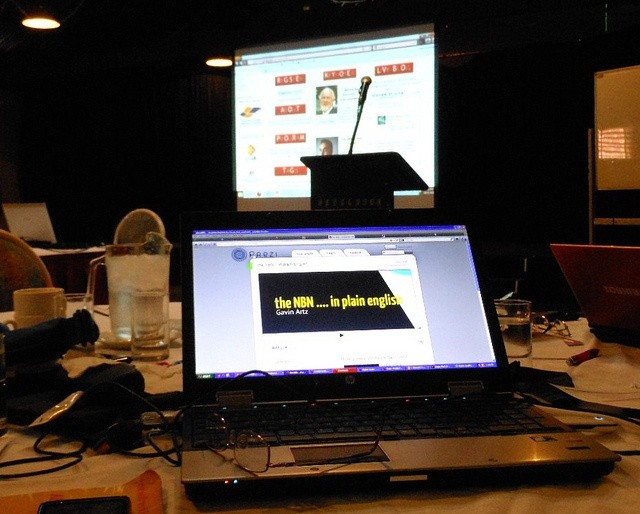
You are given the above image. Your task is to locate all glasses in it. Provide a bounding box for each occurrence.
[532,315,571,338]
[203,412,388,474]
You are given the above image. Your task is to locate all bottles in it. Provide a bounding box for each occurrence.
[0,322,10,436]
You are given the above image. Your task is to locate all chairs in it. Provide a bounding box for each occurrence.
[86,208,165,304]
[0,230,54,311]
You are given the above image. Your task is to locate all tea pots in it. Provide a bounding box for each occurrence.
[80,232,174,341]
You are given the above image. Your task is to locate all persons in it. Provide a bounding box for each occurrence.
[318,140,333,155]
[316,86,337,114]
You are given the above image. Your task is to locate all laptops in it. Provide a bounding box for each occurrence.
[549,229,638,340]
[3,202,62,248]
[176,207,621,493]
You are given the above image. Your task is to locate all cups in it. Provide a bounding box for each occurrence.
[129,286,170,363]
[495,299,533,368]
[52,293,95,352]
[6,287,64,340]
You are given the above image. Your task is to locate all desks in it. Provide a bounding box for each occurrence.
[0,300,639,513]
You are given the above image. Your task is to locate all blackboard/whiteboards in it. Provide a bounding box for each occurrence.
[594,61,640,192]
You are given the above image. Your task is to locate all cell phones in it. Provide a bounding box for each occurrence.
[37,496,131,513]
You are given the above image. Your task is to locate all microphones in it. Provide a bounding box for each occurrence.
[348,77,373,153]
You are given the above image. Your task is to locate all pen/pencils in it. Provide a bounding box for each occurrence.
[567,348,600,366]
